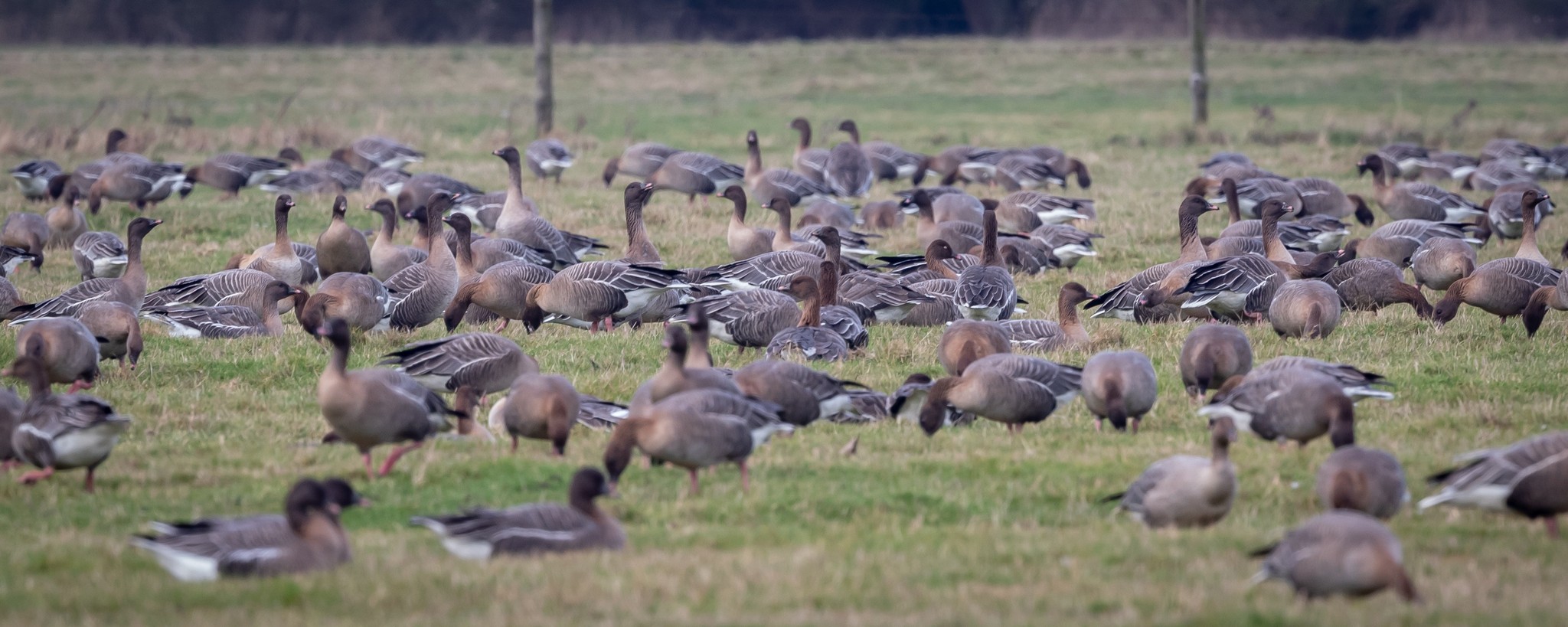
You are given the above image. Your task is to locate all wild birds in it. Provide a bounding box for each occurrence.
[1197,355,1394,451]
[1314,445,1410,518]
[411,466,630,561]
[1083,139,1568,339]
[1246,510,1420,612]
[1,118,1105,495]
[127,478,374,582]
[1080,350,1161,434]
[1417,428,1568,538]
[1101,414,1239,530]
[1178,324,1250,405]
